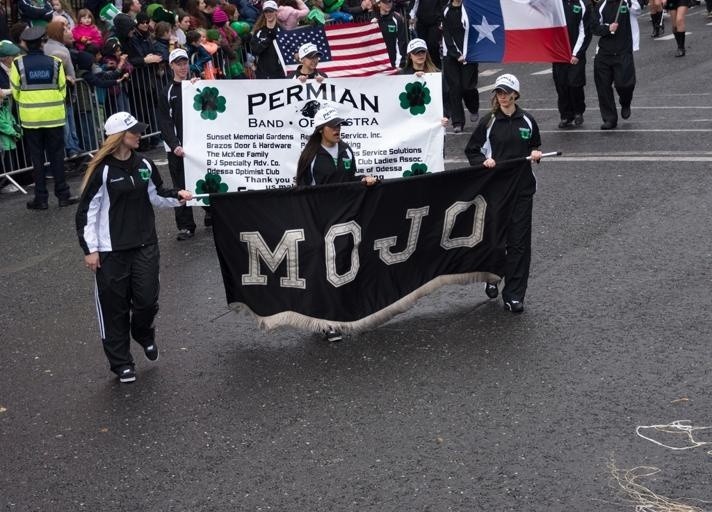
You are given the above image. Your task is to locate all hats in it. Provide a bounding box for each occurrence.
[407,38,427,54]
[20,25,47,43]
[0,40,20,56]
[169,48,189,63]
[299,43,324,61]
[104,111,149,136]
[263,0,278,12]
[492,74,520,100]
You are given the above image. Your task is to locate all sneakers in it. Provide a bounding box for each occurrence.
[120,365,136,382]
[651,30,659,38]
[622,106,631,119]
[144,340,159,362]
[658,25,665,35]
[176,229,195,240]
[559,120,573,128]
[601,122,617,130]
[707,12,712,19]
[676,48,685,57]
[454,126,463,132]
[485,282,499,298]
[326,328,342,342]
[470,114,479,122]
[575,114,583,125]
[204,211,212,226]
[504,300,524,313]
[1,184,18,194]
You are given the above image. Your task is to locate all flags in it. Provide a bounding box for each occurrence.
[461,1,573,63]
[273,20,398,78]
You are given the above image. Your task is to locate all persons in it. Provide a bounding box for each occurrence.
[397,37,449,156]
[9,25,78,209]
[71,1,354,179]
[551,2,710,128]
[300,107,379,343]
[77,111,192,384]
[464,73,542,314]
[2,1,91,191]
[158,48,211,241]
[354,2,480,128]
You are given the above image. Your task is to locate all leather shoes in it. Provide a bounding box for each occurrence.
[26,198,48,210]
[58,195,80,205]
